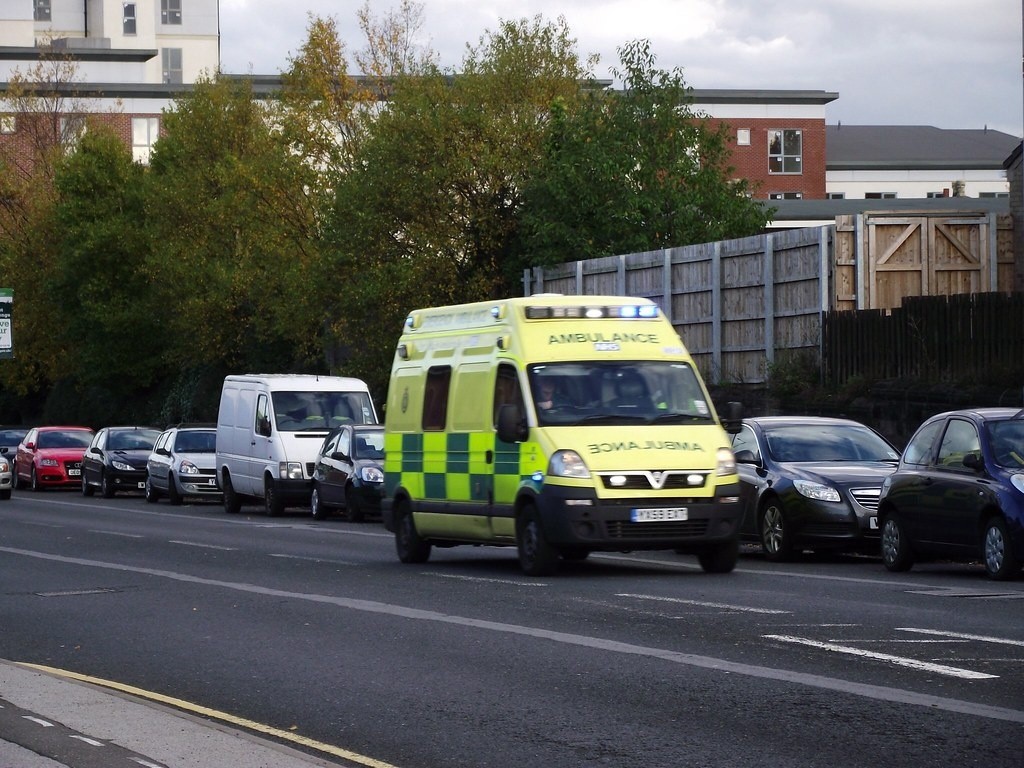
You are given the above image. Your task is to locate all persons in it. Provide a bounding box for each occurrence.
[262,400,285,429]
[536,378,556,411]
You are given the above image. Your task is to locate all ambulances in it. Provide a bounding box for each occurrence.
[381,291,747,578]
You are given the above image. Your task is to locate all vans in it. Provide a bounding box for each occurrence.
[215,372,380,517]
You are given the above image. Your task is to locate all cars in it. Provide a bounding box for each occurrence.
[14,426,97,492]
[310,424,387,524]
[145,423,224,506]
[876,407,1024,580]
[0,429,31,472]
[80,426,164,498]
[0,447,13,499]
[723,415,902,562]
